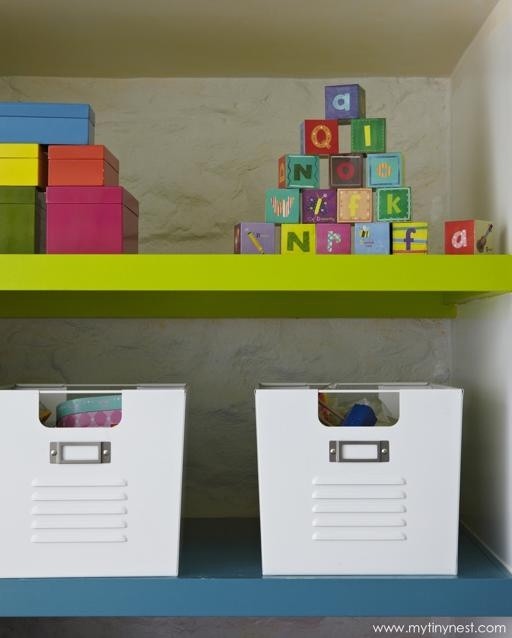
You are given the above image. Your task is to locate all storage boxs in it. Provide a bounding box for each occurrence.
[0,101,95,144]
[46,186,139,253]
[0,186,46,253]
[0,143,47,190]
[254,382,464,576]
[48,144,119,186]
[1,384,189,577]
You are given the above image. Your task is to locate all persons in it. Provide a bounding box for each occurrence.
[233,223,275,253]
[368,153,403,189]
[354,222,391,254]
[445,220,496,254]
[302,189,337,224]
[316,223,352,254]
[278,154,321,190]
[324,84,367,125]
[265,188,301,224]
[350,119,386,156]
[282,223,316,254]
[377,187,411,222]
[328,153,364,188]
[337,189,374,224]
[392,222,429,254]
[300,119,339,159]
